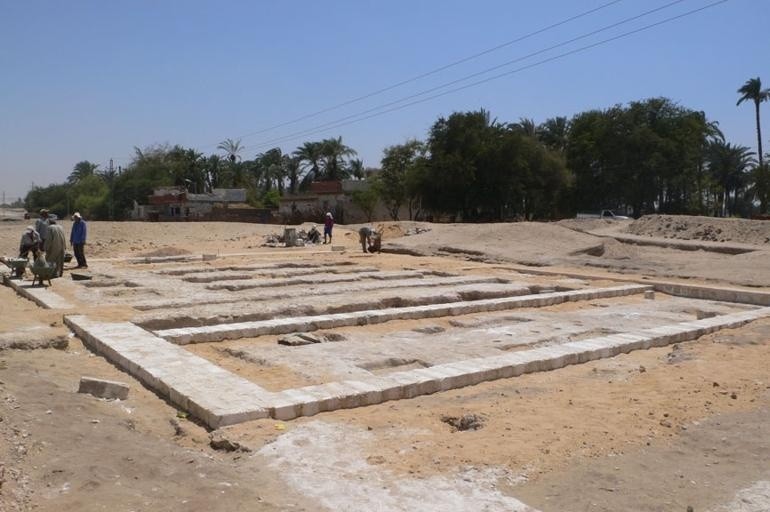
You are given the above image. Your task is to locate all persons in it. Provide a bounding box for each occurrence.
[359,227,377,253]
[306,224,322,244]
[367,231,381,254]
[322,212,335,245]
[40,214,66,278]
[15,226,40,280]
[69,211,88,269]
[36,208,52,252]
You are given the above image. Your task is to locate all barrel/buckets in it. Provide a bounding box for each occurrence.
[285,228,296,247]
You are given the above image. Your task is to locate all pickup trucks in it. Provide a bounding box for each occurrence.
[577,209,635,220]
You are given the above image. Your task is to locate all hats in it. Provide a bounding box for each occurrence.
[72,212,81,218]
[26,226,34,233]
[40,209,49,214]
